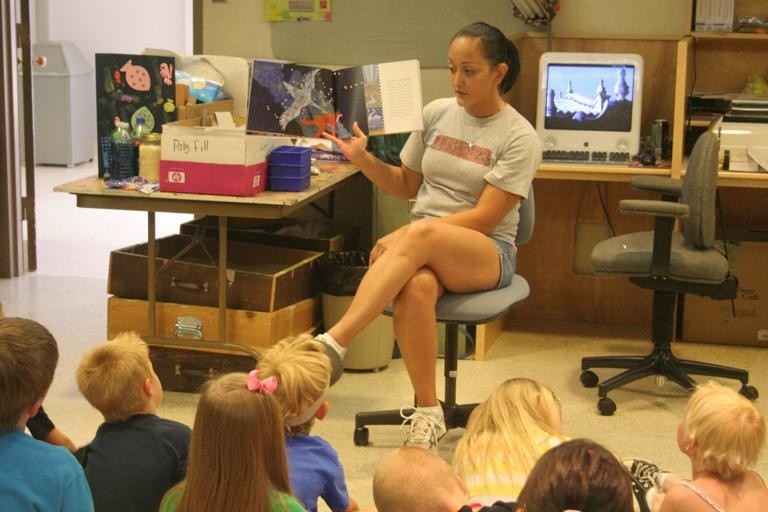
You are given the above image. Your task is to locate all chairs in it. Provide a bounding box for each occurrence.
[354,183,536,447]
[581,131,757,416]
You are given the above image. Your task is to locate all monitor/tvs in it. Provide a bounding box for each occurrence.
[535,50,644,157]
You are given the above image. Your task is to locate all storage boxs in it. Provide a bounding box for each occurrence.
[159,99,295,197]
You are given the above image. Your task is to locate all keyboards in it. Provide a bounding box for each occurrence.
[540,151,630,165]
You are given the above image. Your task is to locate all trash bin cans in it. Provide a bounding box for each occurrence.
[316,250,395,373]
[18,40,96,168]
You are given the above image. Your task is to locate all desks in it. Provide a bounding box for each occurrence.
[54,161,361,393]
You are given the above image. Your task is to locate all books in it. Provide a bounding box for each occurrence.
[245,59,425,139]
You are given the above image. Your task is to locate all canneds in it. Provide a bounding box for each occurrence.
[651,119,670,162]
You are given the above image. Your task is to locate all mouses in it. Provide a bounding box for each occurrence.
[642,152,657,166]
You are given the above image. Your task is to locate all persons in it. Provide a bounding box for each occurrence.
[516,439,633,512]
[0,316,95,511]
[255,333,360,512]
[451,378,572,512]
[622,379,768,512]
[0,298,76,453]
[74,332,193,512]
[159,369,308,512]
[313,21,543,452]
[373,446,517,512]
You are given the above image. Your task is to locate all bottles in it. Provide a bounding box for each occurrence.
[132,118,150,176]
[138,132,160,185]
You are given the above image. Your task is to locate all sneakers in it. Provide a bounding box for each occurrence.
[626,470,663,512]
[400,399,448,454]
[620,458,672,494]
